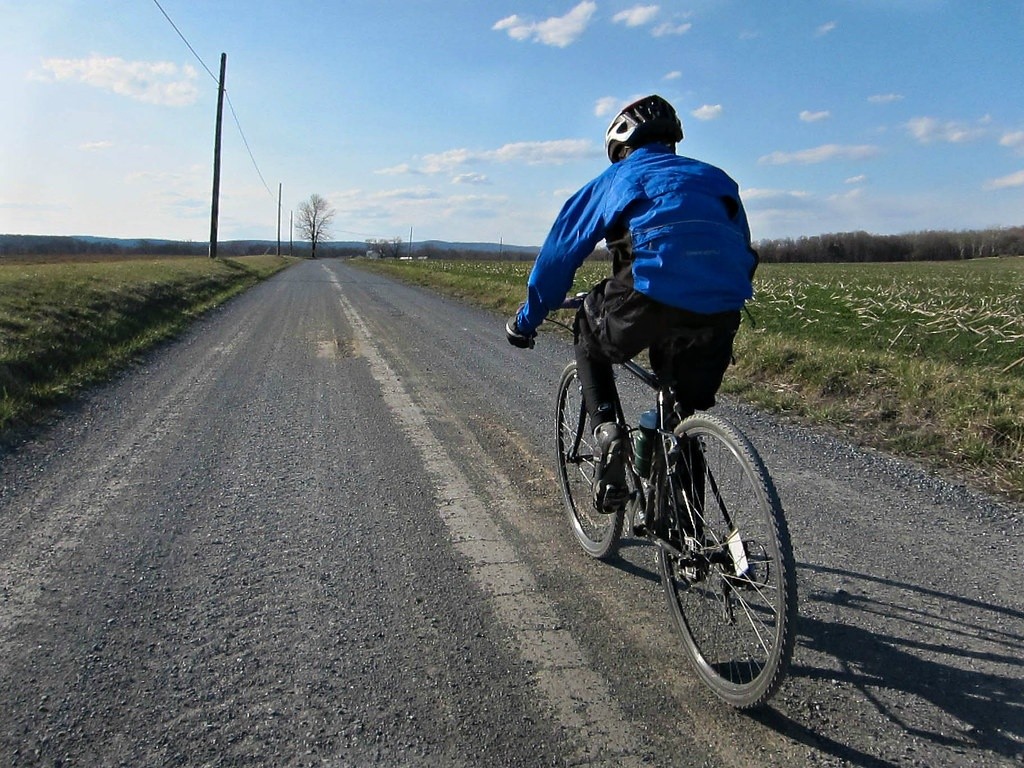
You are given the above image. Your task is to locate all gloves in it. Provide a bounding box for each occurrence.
[505,315,537,349]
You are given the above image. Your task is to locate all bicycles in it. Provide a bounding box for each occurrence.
[515,290,798,711]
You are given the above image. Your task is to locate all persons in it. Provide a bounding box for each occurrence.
[506,94,761,535]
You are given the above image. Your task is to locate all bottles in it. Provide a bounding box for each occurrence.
[633,408,654,479]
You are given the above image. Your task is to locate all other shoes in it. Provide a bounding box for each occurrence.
[591,422,626,514]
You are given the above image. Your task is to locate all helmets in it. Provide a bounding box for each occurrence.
[605,94,683,164]
